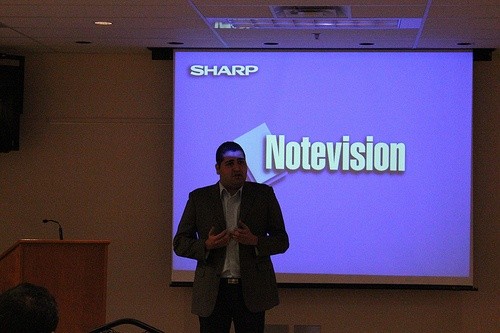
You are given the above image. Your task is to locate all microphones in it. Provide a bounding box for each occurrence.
[42,219,64,240]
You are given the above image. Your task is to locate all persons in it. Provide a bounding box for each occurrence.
[0,283,60,333]
[172,141,289,333]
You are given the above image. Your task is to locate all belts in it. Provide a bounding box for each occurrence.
[220,277,240,284]
